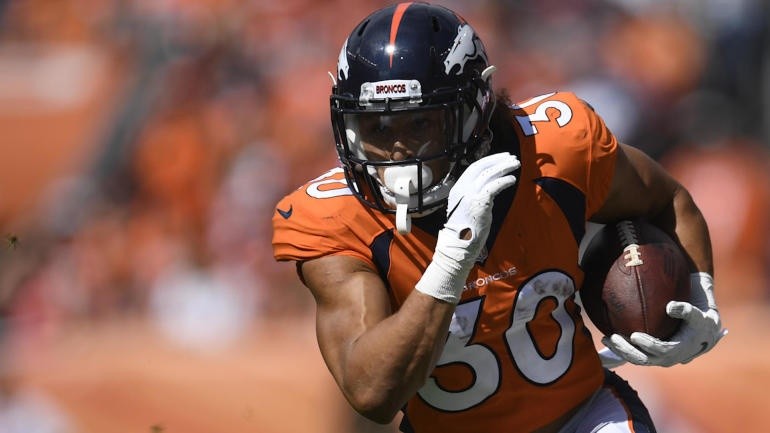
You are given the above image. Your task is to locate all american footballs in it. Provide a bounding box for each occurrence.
[576,216,694,350]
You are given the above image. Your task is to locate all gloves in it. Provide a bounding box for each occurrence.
[413,152,521,306]
[602,271,728,368]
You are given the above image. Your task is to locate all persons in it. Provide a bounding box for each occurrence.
[272,0,724,433]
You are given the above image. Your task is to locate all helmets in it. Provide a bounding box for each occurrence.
[328,1,496,219]
